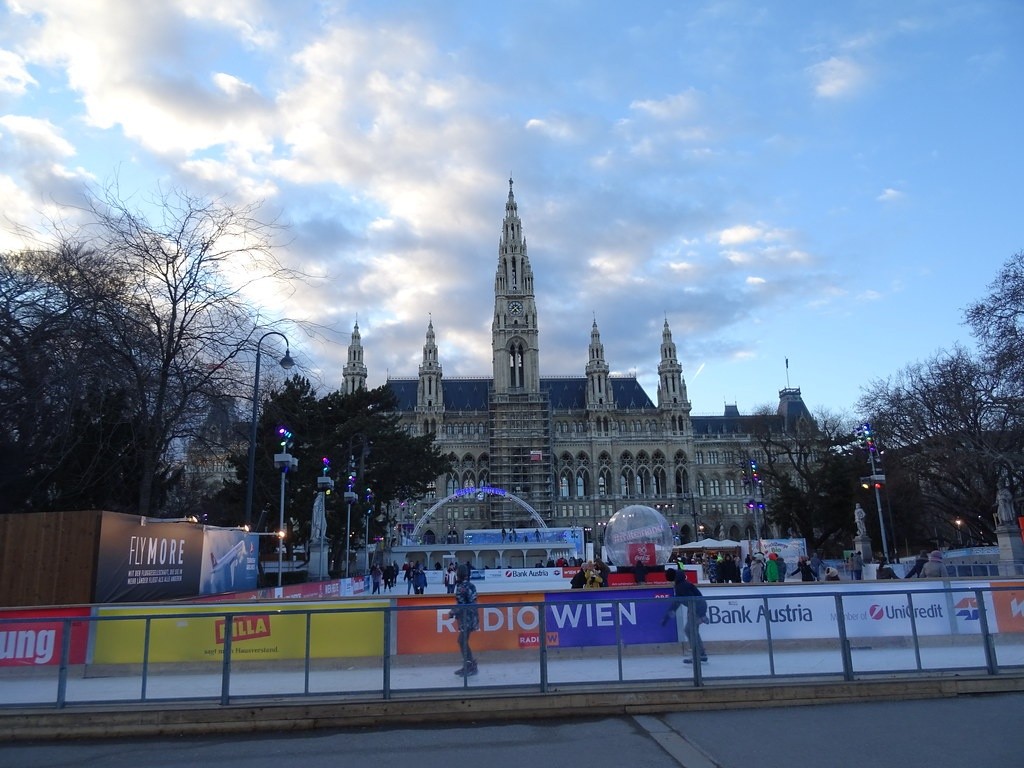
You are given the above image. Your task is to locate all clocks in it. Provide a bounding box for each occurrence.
[508,301,524,317]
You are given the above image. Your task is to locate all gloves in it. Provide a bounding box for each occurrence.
[661,615,669,625]
[701,616,710,625]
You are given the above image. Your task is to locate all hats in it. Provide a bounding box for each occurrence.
[931,550,942,559]
[665,568,676,581]
[754,554,763,561]
[921,550,927,556]
[456,564,470,579]
[801,556,809,561]
[717,556,723,561]
[879,557,887,563]
[581,563,588,569]
[418,563,423,568]
[769,553,777,560]
[587,561,595,567]
[824,567,838,578]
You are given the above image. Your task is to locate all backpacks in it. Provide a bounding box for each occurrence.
[743,567,753,582]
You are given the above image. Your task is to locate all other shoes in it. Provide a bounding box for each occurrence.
[460,661,477,677]
[700,654,708,662]
[455,667,463,675]
[683,658,694,664]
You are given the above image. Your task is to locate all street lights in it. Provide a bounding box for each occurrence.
[345,490,358,577]
[657,505,675,517]
[683,493,699,542]
[598,522,606,557]
[585,528,591,563]
[274,454,299,585]
[744,498,763,553]
[366,507,372,574]
[318,477,335,580]
[861,475,889,564]
[246,330,296,527]
[570,526,578,547]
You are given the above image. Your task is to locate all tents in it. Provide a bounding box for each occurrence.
[673,538,743,549]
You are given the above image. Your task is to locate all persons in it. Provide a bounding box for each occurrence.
[500,526,518,544]
[570,558,610,589]
[993,481,1015,525]
[309,491,332,540]
[540,555,583,567]
[877,547,901,564]
[445,562,458,593]
[391,534,396,547]
[903,550,929,580]
[631,561,648,586]
[848,551,865,580]
[920,550,948,577]
[370,559,400,595]
[402,560,428,595]
[465,561,474,582]
[661,569,709,663]
[533,528,541,543]
[417,536,423,545]
[455,562,459,570]
[443,562,481,677]
[434,561,442,570]
[853,502,867,536]
[669,551,822,583]
